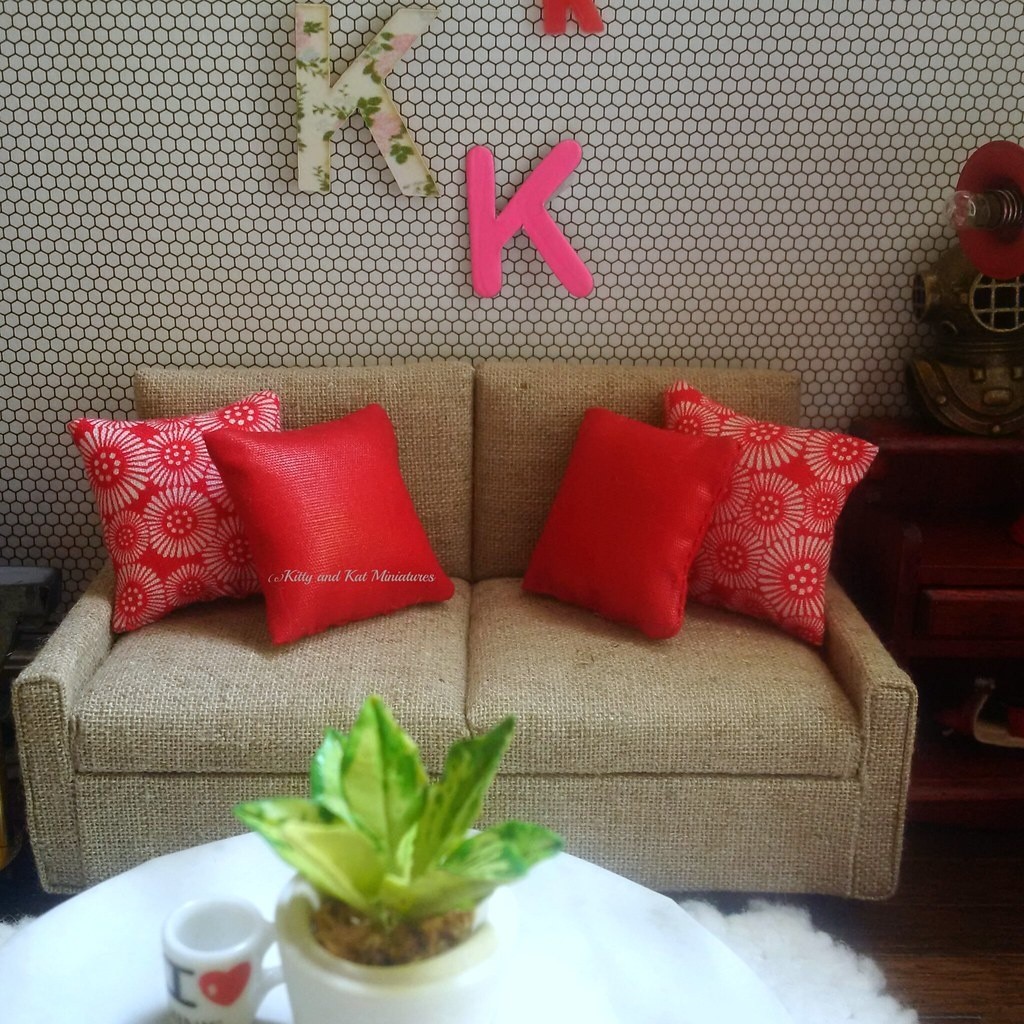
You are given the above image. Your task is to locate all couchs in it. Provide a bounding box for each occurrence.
[7,366,921,908]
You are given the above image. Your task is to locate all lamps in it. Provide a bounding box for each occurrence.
[942,140,1023,281]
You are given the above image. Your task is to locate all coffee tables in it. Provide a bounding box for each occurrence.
[1,810,779,1022]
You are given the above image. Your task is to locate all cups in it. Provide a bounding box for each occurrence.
[164,898,296,1024]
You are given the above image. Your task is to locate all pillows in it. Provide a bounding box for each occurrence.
[203,403,455,647]
[63,387,287,634]
[646,380,877,648]
[521,407,744,642]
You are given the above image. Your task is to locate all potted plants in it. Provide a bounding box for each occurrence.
[229,694,566,1024]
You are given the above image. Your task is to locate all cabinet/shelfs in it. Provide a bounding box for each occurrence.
[843,418,1022,824]
